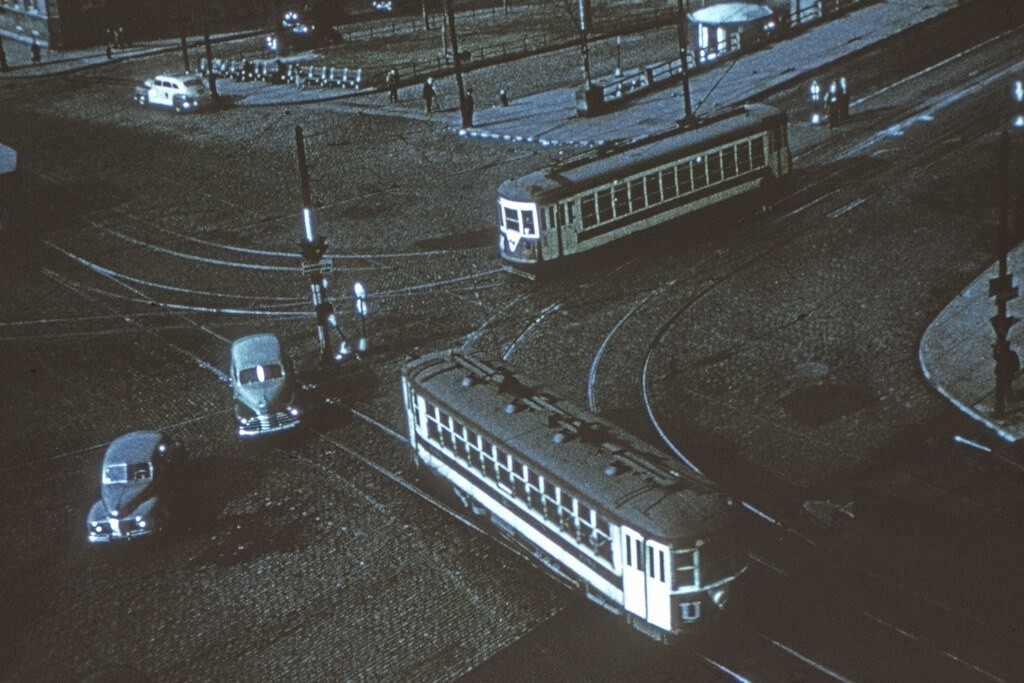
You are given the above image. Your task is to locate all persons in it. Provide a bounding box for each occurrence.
[422,75,436,116]
[385,68,399,104]
[133,464,147,480]
[464,88,475,127]
[823,77,850,129]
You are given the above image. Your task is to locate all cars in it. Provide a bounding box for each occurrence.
[134,73,213,114]
[229,333,302,438]
[86,430,187,547]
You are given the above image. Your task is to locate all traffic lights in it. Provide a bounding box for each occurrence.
[1005,72,1024,139]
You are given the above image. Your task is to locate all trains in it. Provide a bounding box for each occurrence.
[400,228,752,647]
[496,56,792,280]
